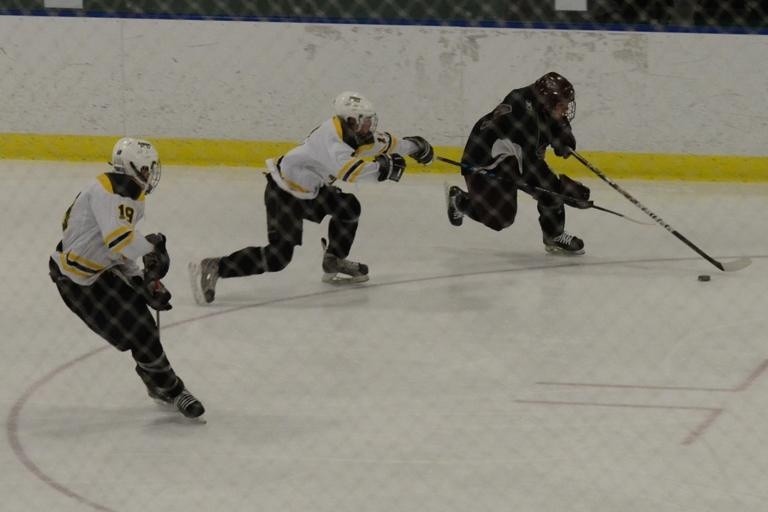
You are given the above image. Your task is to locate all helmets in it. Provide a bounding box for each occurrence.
[112,137,160,195]
[334,91,378,144]
[534,72,576,125]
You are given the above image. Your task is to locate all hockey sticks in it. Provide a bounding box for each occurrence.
[436,154,655,226]
[565,145,753,273]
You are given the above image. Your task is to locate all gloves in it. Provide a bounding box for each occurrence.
[129,233,172,311]
[375,153,405,182]
[553,131,576,159]
[559,173,593,208]
[402,136,434,166]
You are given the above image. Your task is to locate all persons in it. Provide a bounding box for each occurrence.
[48,137,205,418]
[198,91,434,304]
[446,71,593,251]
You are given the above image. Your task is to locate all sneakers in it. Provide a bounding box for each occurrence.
[447,186,463,225]
[147,386,204,418]
[202,257,222,302]
[542,230,584,251]
[321,250,368,276]
